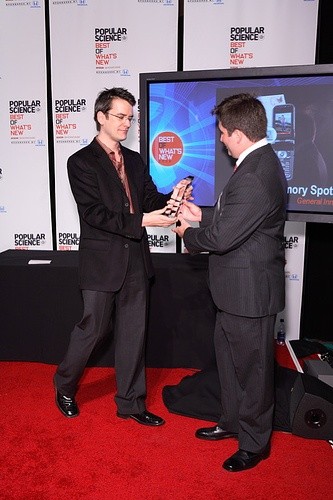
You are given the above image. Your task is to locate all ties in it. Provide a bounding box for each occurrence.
[232,166,237,172]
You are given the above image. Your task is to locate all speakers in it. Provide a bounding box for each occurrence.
[289,373,333,441]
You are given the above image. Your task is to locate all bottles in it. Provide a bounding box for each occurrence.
[276,318,286,346]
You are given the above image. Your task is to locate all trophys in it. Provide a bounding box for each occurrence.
[165,176,193,218]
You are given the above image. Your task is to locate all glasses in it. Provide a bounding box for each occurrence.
[105,112,136,123]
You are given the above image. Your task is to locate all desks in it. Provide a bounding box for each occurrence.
[0,249,216,368]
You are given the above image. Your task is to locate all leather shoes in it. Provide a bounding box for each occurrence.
[117,410,164,425]
[196,424,238,439]
[223,448,269,472]
[55,385,78,417]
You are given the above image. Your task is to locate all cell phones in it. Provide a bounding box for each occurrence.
[271,104,296,181]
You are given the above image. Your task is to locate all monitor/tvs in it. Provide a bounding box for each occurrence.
[139,64,333,223]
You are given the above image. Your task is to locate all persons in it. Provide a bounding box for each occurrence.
[53,87,195,426]
[172,94,287,471]
